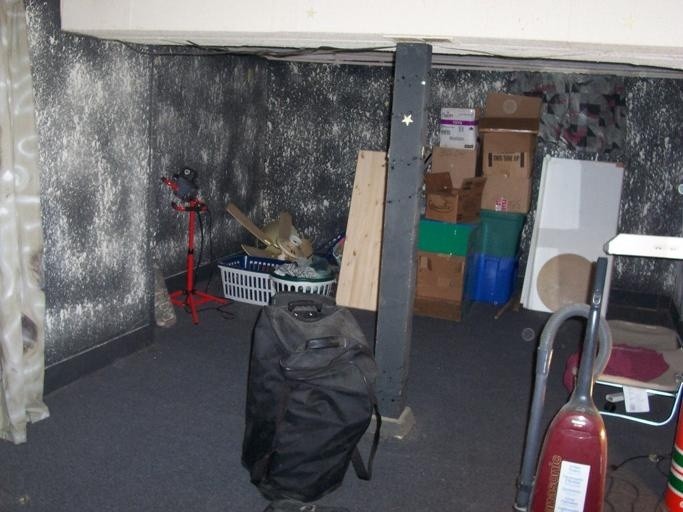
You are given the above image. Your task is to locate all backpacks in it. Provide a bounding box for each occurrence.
[241,290,383,504]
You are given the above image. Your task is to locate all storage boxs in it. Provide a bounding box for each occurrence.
[411,92,543,320]
